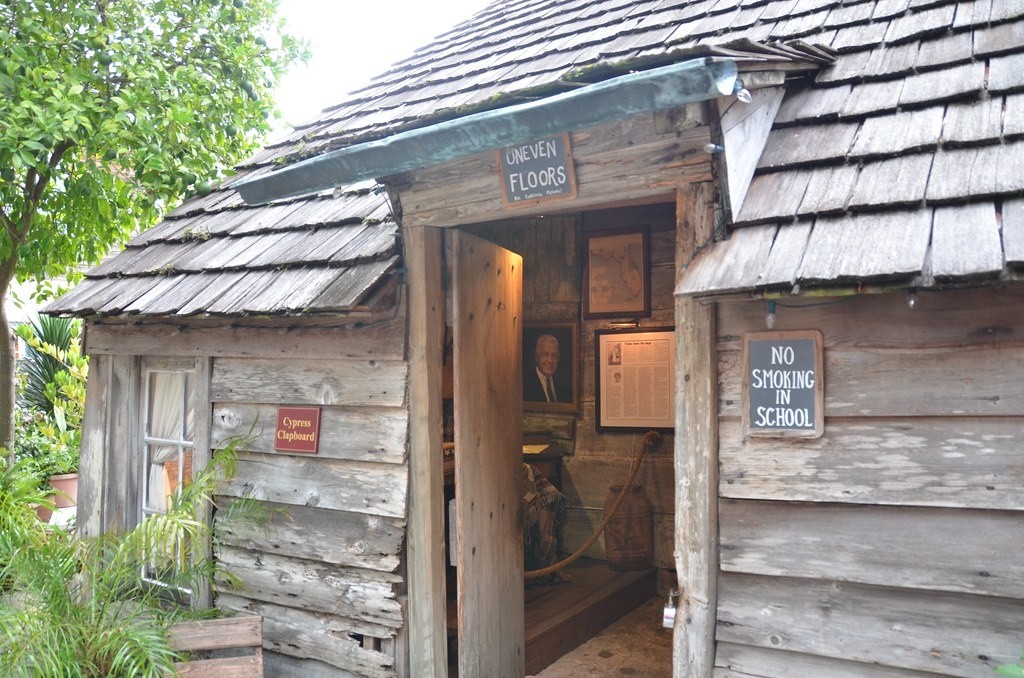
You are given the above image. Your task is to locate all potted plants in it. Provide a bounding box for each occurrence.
[14,439,80,522]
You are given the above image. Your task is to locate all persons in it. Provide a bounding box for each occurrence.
[523,335,573,403]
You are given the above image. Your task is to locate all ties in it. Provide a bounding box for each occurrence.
[546,377,556,404]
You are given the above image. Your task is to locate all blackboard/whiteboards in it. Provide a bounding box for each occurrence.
[742,329,825,440]
[494,131,577,210]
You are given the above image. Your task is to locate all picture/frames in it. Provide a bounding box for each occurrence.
[594,326,675,433]
[580,225,651,320]
[522,321,582,415]
